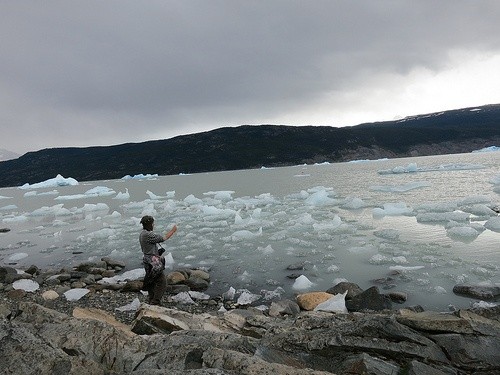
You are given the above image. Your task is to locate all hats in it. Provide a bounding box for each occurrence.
[142,216,154,224]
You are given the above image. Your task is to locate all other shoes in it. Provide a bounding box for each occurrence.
[148,301,161,306]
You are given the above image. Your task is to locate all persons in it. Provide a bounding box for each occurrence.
[139,215,178,306]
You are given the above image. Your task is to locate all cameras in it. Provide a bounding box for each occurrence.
[158,248,165,256]
